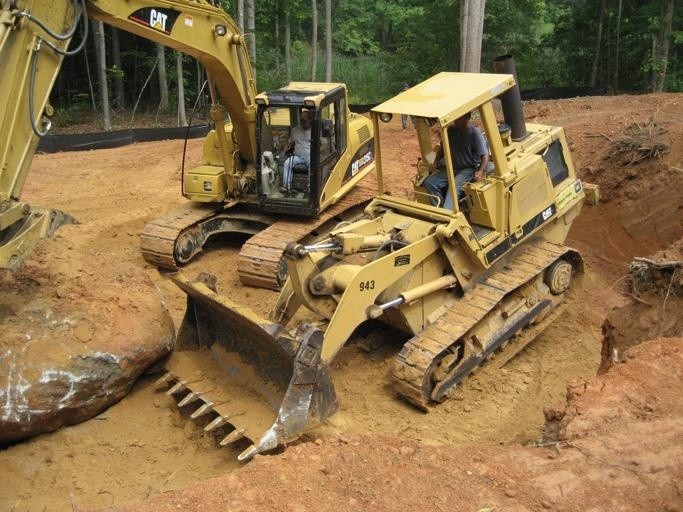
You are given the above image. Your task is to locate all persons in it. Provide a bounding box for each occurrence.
[279,111,328,196]
[425,111,489,209]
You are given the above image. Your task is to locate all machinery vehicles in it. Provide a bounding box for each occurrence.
[149,53,603,465]
[0,0,392,294]
